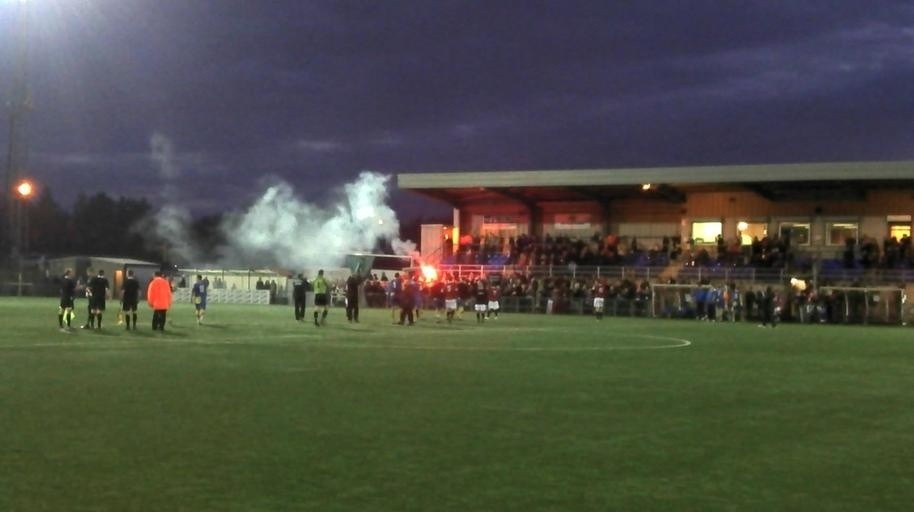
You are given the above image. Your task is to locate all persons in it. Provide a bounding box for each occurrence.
[500,277,858,323]
[692,229,914,274]
[458,225,686,268]
[257,269,503,325]
[58,265,238,331]
